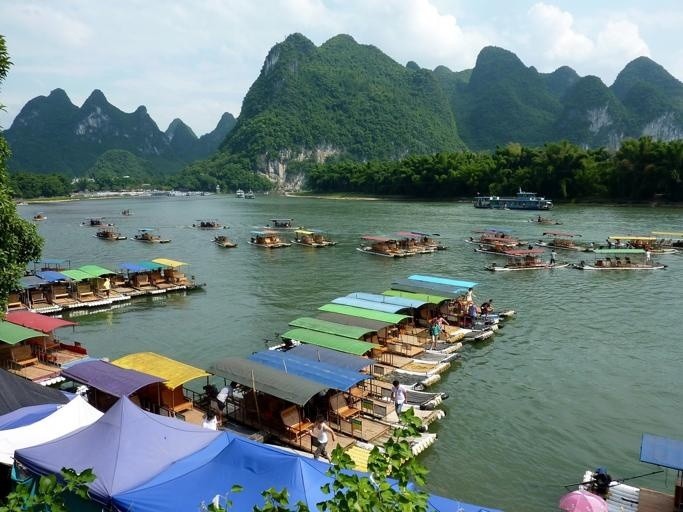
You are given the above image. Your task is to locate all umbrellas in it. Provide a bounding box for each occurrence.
[558,490,609,512]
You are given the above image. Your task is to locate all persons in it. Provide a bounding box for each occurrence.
[201,410,218,431]
[425,288,492,350]
[495,216,683,266]
[102,277,110,297]
[588,468,611,501]
[216,381,237,426]
[314,415,335,463]
[391,381,407,423]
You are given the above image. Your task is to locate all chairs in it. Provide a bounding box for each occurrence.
[37,331,60,355]
[329,383,367,425]
[9,345,38,371]
[4,268,187,312]
[280,405,314,442]
[160,385,192,417]
[365,335,387,358]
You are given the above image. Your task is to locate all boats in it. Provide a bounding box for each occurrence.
[0,258,206,386]
[189,217,229,230]
[465,230,683,270]
[212,235,237,247]
[358,230,446,258]
[82,209,172,243]
[235,189,255,199]
[270,275,516,409]
[580,431,683,512]
[472,188,554,212]
[246,218,339,249]
[32,213,47,221]
